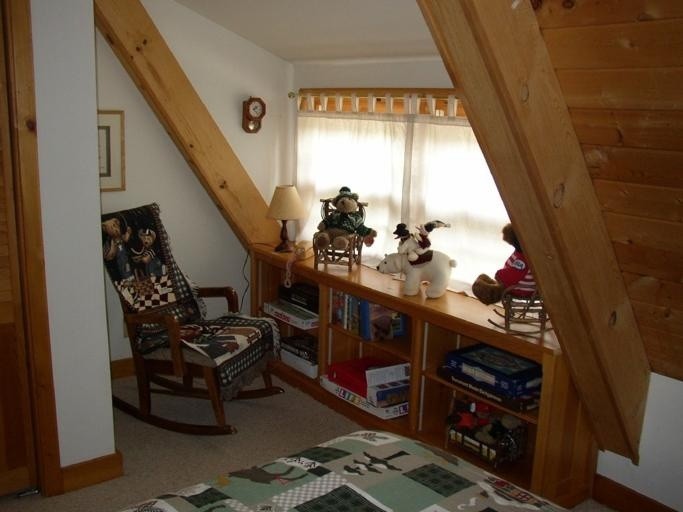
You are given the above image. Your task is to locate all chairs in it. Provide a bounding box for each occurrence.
[99,201,285,437]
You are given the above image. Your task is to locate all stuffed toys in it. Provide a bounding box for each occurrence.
[376,220,457,298]
[314,186,377,251]
[472,223,537,307]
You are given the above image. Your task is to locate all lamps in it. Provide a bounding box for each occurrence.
[264,184,307,253]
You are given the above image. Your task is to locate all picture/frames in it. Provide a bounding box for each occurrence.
[96,110,125,192]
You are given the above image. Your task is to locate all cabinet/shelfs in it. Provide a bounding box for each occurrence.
[246,240,598,509]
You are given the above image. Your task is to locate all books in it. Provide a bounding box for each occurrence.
[333,291,402,341]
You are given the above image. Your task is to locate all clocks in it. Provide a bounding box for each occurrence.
[242,96,265,134]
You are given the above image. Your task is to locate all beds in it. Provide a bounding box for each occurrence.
[113,427,568,512]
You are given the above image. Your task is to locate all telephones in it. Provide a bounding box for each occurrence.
[294,241,315,260]
